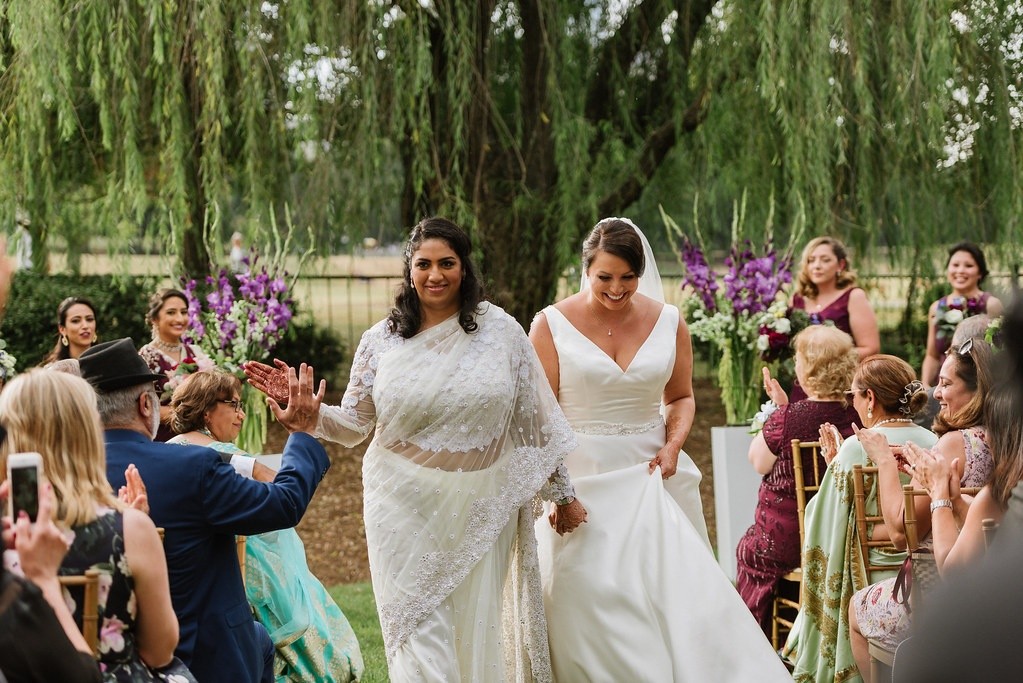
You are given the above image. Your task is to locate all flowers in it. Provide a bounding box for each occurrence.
[930,294,969,340]
[0,348,17,382]
[984,316,1009,352]
[755,284,834,363]
[659,181,806,426]
[160,357,218,402]
[167,198,318,456]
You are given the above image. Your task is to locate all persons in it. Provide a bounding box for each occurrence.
[737,237,1023,683]
[528,217,796,683]
[0,231,364,683]
[243,218,588,683]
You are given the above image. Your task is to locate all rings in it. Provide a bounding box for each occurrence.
[912,464,916,470]
[822,444,826,449]
[764,385,769,388]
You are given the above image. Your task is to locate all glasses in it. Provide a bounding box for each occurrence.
[216,399,244,413]
[958,336,983,378]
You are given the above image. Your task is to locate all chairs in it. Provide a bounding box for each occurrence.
[771,439,1001,652]
[58,568,99,660]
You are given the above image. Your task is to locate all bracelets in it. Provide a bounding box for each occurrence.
[930,500,953,513]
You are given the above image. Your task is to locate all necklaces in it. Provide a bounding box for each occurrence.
[871,418,912,428]
[153,336,182,352]
[816,291,834,309]
[590,295,632,336]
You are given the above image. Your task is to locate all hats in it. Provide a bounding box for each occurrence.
[75,337,167,395]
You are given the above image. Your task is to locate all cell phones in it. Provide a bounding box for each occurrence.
[6,452,44,532]
